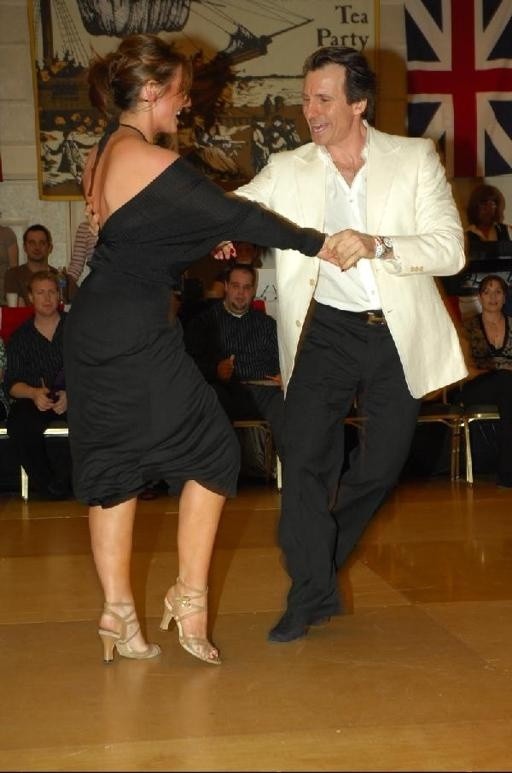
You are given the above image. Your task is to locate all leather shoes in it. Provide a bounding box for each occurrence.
[270,612,330,641]
[139,482,169,501]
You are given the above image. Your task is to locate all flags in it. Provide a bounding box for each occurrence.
[404,0,512,183]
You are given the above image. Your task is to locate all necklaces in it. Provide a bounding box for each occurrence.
[118,123,149,144]
[486,317,502,337]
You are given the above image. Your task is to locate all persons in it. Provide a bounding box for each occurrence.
[462,185,512,257]
[457,274,512,488]
[237,76,255,90]
[62,32,359,667]
[84,44,470,640]
[64,221,99,283]
[2,271,70,501]
[41,94,303,189]
[0,227,19,279]
[185,262,285,451]
[0,337,16,423]
[3,224,71,306]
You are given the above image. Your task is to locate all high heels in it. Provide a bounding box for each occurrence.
[98,601,161,662]
[160,576,222,664]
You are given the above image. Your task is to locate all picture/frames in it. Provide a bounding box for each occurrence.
[26,0,382,202]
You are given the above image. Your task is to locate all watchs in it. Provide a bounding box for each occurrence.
[374,235,393,259]
